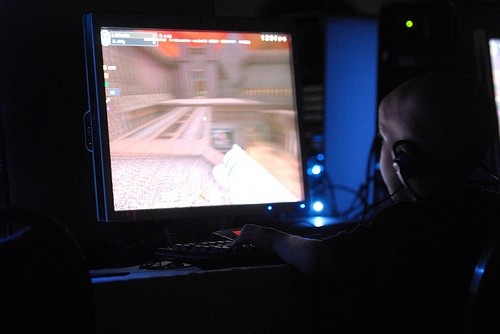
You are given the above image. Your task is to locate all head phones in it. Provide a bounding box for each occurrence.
[391,139,500,210]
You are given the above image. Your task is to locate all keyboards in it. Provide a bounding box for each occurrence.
[155,233,341,265]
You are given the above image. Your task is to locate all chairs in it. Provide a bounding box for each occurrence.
[462,233,500,334]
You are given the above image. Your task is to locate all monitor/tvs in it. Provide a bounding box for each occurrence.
[83,11,310,243]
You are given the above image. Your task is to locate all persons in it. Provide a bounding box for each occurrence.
[229,72,500,334]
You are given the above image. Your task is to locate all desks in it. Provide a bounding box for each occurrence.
[89,260,293,284]
